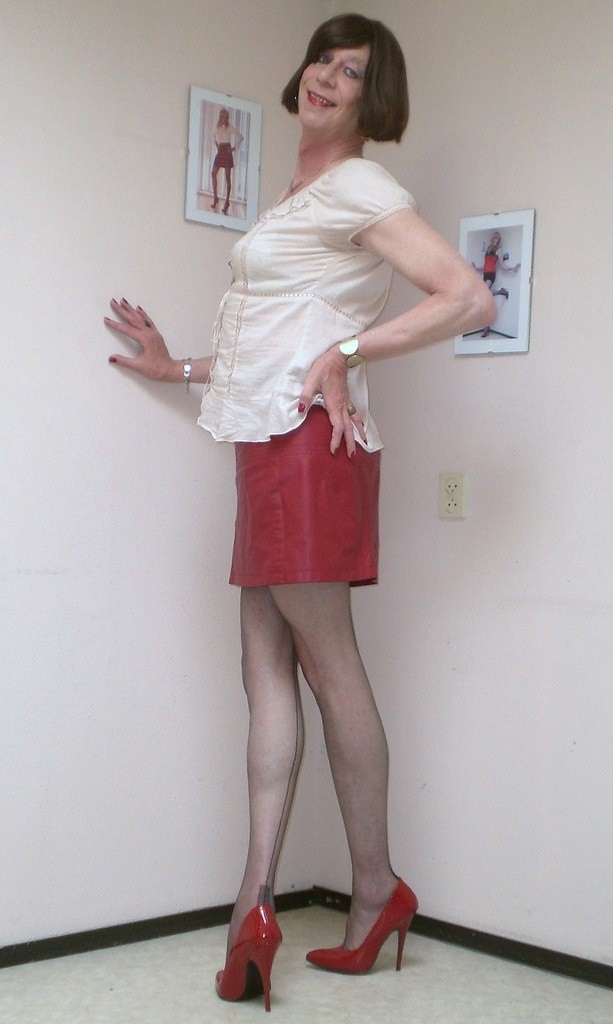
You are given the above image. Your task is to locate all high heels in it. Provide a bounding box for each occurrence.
[500,288,509,299]
[221,203,230,212]
[211,198,219,207]
[481,327,490,337]
[216,902,283,1012]
[306,878,417,975]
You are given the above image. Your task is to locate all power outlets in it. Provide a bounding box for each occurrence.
[438,472,465,518]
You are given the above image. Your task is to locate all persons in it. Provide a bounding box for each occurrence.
[210,108,245,212]
[102,11,499,1011]
[472,232,521,337]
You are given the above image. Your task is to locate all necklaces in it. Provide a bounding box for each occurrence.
[287,149,361,193]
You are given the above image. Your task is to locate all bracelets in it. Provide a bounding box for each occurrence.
[338,336,367,370]
[184,358,190,391]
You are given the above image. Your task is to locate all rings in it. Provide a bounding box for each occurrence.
[347,403,354,413]
[143,320,150,328]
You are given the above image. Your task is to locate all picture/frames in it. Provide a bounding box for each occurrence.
[184,84,262,233]
[454,208,535,356]
